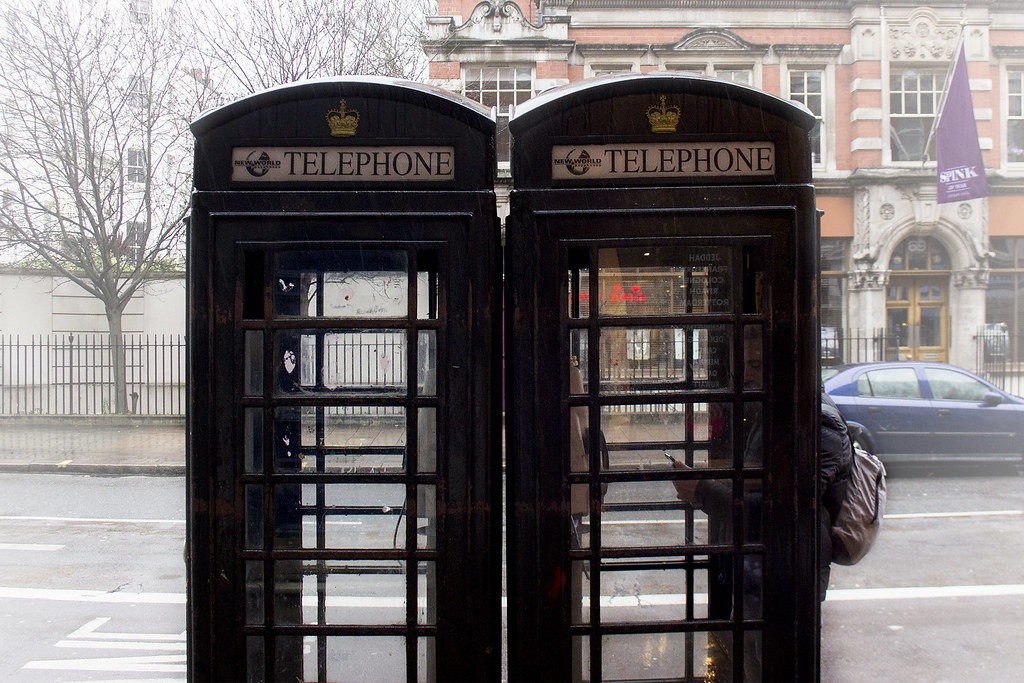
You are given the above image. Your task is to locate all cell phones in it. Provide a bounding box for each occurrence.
[664,452,675,463]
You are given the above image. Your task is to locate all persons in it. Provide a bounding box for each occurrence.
[672,324,852,683]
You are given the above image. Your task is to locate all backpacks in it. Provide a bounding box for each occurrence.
[822,403,888,565]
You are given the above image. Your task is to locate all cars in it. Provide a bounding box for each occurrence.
[823,361,1024,466]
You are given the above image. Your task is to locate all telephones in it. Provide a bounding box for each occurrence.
[401,369,436,519]
[570,364,611,518]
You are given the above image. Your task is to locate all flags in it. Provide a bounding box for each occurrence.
[935,41,990,204]
